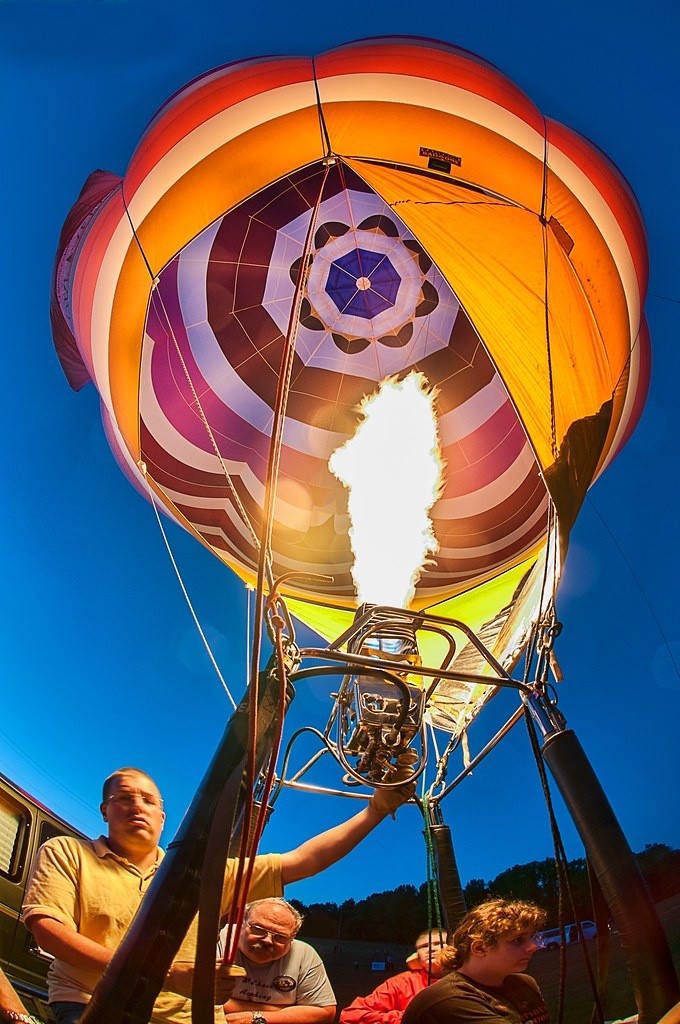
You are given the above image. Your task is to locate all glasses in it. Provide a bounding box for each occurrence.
[245,918,294,947]
[105,791,164,812]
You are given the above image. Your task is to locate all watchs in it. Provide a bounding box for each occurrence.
[252,1009,266,1024]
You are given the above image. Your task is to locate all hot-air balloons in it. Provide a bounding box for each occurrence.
[51,33,679,1024]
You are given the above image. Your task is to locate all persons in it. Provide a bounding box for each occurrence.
[224,897,337,1024]
[400,899,680,1024]
[0,967,44,1024]
[339,928,449,1024]
[21,759,417,1024]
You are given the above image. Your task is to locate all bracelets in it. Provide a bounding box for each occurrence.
[0,1010,39,1024]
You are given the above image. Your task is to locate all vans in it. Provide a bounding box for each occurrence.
[532,920,597,952]
